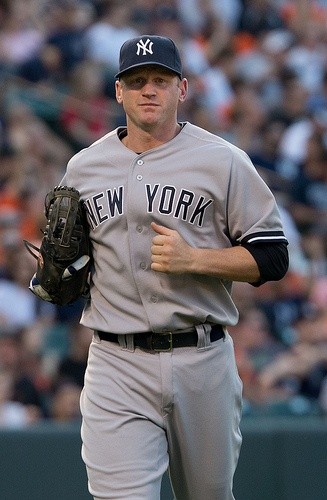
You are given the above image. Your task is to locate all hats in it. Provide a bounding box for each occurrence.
[114,36,184,79]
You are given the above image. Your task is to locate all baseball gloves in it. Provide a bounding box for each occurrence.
[23,186,91,307]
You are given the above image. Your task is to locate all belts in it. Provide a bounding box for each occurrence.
[95,325,225,350]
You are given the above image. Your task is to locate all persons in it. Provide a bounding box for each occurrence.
[0,1,327,430]
[23,36,289,500]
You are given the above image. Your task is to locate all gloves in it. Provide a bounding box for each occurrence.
[29,254,90,306]
